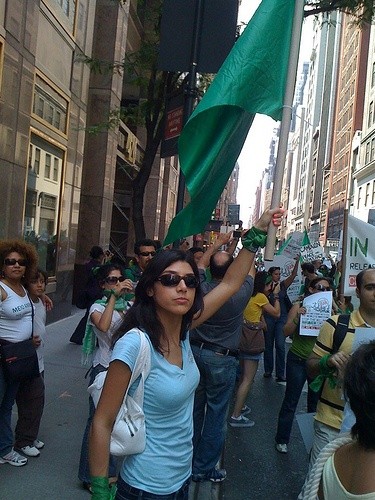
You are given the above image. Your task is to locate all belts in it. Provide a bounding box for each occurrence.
[191,339,238,359]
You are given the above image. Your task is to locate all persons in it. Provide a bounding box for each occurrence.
[69,226,375,500]
[87,202,284,500]
[0,239,53,468]
[22,268,47,448]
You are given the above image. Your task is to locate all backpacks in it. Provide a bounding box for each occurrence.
[72,261,98,309]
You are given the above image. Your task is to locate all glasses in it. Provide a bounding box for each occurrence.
[3,258,27,267]
[156,274,201,289]
[313,285,331,292]
[139,251,158,256]
[105,276,126,285]
[270,267,281,271]
[320,268,325,270]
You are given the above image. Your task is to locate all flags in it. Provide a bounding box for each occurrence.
[161,0,303,249]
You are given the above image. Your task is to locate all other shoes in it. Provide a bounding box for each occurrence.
[70,339,83,345]
[277,444,288,453]
[264,374,271,377]
[275,378,287,382]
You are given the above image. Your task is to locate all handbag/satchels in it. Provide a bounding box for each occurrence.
[241,319,266,354]
[0,339,40,384]
[87,328,151,456]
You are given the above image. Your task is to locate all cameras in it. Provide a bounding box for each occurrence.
[106,250,113,256]
[233,229,241,237]
[125,282,138,293]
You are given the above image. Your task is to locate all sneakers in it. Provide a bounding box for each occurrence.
[0,451,28,466]
[192,470,226,482]
[12,446,40,457]
[240,406,251,414]
[230,416,255,427]
[34,439,45,449]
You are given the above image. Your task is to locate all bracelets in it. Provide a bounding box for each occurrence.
[90,476,116,500]
[346,308,351,314]
[293,318,298,326]
[309,354,341,392]
[104,289,119,299]
[274,296,279,299]
[337,308,342,315]
[241,226,267,253]
